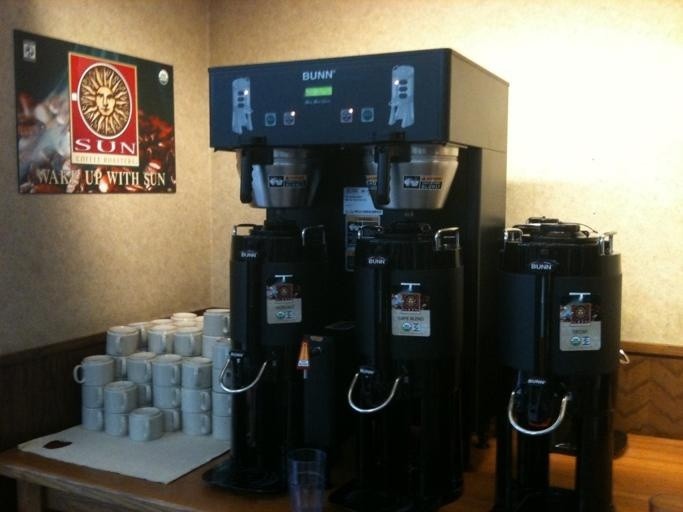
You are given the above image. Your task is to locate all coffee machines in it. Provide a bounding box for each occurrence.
[201,50,507,510]
[493,216,621,510]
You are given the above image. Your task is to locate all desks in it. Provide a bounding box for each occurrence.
[0,422,683,512]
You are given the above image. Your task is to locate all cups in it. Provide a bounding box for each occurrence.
[285,446,326,512]
[72,308,231,440]
